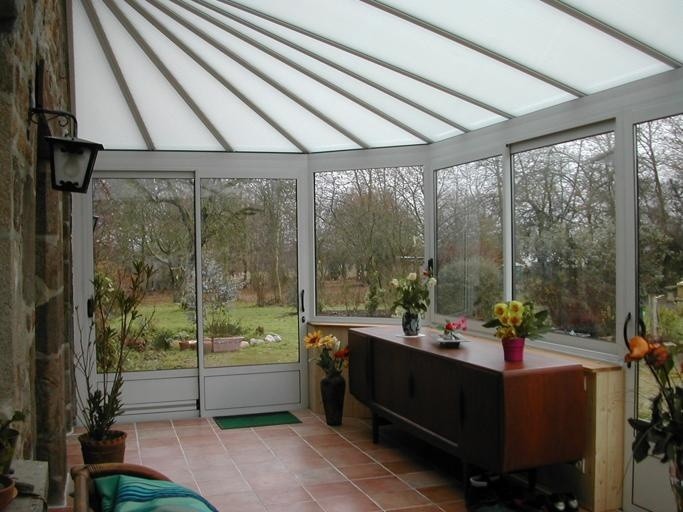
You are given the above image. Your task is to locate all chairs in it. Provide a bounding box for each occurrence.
[70,462,174,512]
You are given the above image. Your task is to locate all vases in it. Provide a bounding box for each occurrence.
[502,336,525,361]
[320,374,346,427]
[443,328,453,340]
[402,311,422,336]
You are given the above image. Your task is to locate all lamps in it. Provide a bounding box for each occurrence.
[28,106,104,194]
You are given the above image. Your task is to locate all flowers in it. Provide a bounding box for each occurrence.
[481,299,553,342]
[389,271,437,319]
[436,313,470,332]
[303,328,351,377]
[624,334,683,512]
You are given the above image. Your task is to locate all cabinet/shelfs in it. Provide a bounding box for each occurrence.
[347,325,589,512]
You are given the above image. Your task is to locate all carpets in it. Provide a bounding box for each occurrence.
[212,410,303,430]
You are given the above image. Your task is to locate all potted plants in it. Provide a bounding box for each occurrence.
[0,412,18,506]
[0,407,33,475]
[70,255,162,465]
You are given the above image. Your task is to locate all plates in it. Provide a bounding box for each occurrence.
[430,332,471,343]
